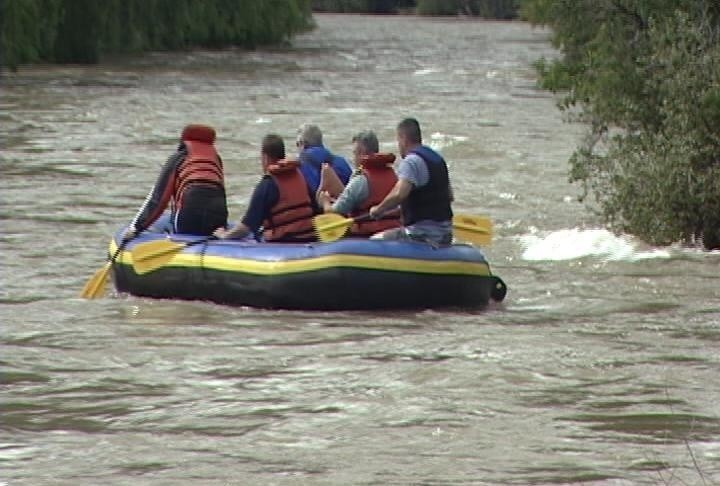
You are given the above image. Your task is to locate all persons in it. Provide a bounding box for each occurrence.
[293,122,352,202]
[316,128,400,236]
[210,133,319,243]
[368,117,453,247]
[119,124,230,240]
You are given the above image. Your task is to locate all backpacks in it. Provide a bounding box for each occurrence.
[299,148,345,207]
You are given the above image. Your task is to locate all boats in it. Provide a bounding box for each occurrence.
[106,213,507,313]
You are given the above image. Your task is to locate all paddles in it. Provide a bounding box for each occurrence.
[132,235,220,275]
[453,216,492,245]
[313,212,373,242]
[81,236,127,299]
